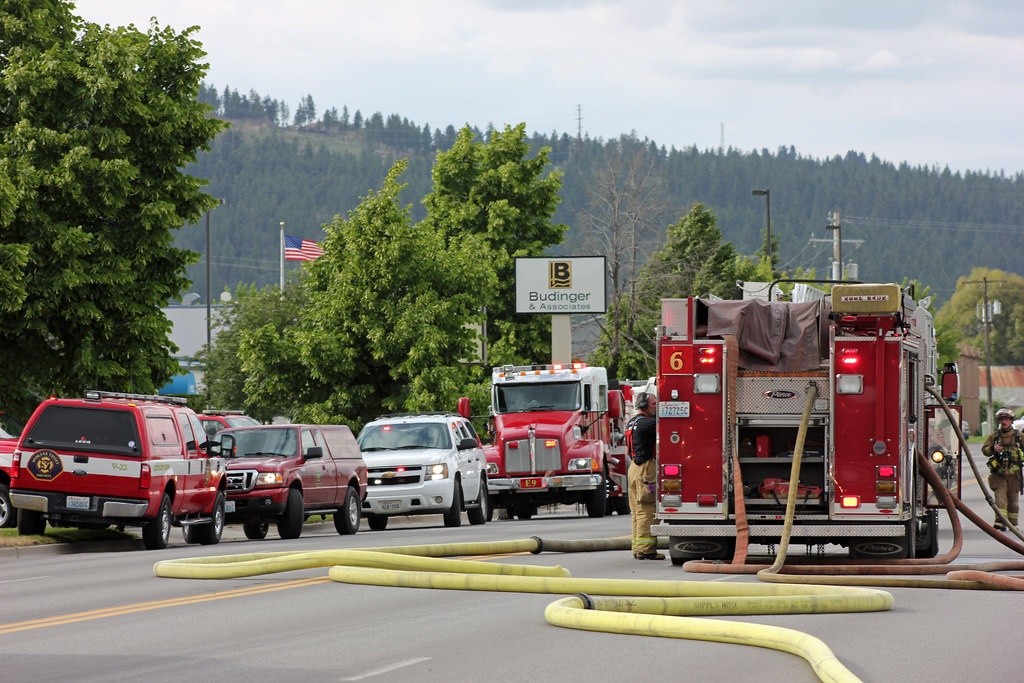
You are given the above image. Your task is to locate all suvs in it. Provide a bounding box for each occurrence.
[181,424,368,544]
[8,389,229,552]
[356,411,489,531]
[192,409,262,440]
[0,411,20,527]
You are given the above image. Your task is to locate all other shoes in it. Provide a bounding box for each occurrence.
[636,553,665,560]
[993,521,1007,531]
[634,554,637,559]
[1010,525,1019,531]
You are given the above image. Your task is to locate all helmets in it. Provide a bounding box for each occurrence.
[996,408,1014,419]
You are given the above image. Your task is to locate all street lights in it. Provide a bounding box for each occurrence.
[752,189,771,257]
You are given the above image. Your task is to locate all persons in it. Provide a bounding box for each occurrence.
[981,408,1024,531]
[626,392,666,560]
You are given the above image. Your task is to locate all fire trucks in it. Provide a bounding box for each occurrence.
[456,364,656,521]
[653,281,963,565]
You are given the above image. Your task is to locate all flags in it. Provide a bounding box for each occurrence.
[284,234,325,261]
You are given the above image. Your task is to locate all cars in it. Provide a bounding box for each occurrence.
[1012,416,1022,433]
[962,420,969,440]
